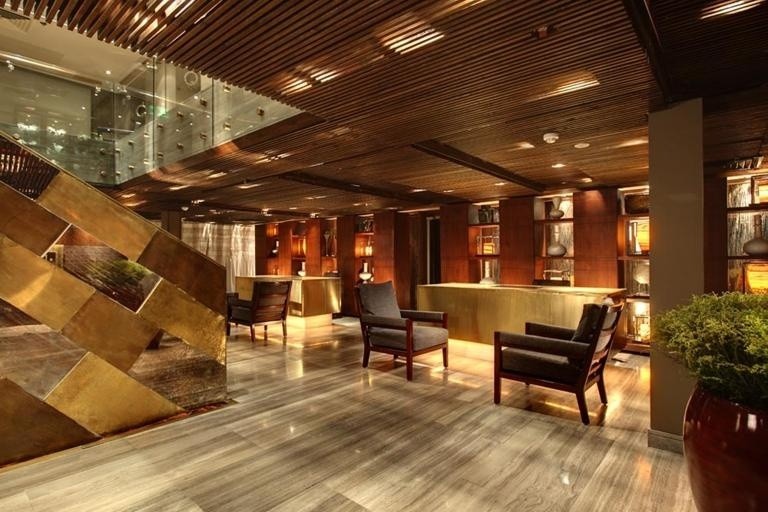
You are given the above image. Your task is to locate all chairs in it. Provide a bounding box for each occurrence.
[227,280,292,342]
[352,286,449,381]
[494,299,624,426]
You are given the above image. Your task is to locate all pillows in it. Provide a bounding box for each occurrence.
[358,281,402,319]
[568,298,615,365]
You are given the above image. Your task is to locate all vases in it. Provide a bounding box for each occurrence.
[744,215,768,256]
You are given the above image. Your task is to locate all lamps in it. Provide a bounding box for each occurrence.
[742,260,768,293]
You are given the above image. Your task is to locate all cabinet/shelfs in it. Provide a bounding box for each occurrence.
[440,184,650,355]
[719,167,768,291]
[255,211,411,318]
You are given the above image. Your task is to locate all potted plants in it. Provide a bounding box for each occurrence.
[85,259,145,311]
[650,288,768,512]
